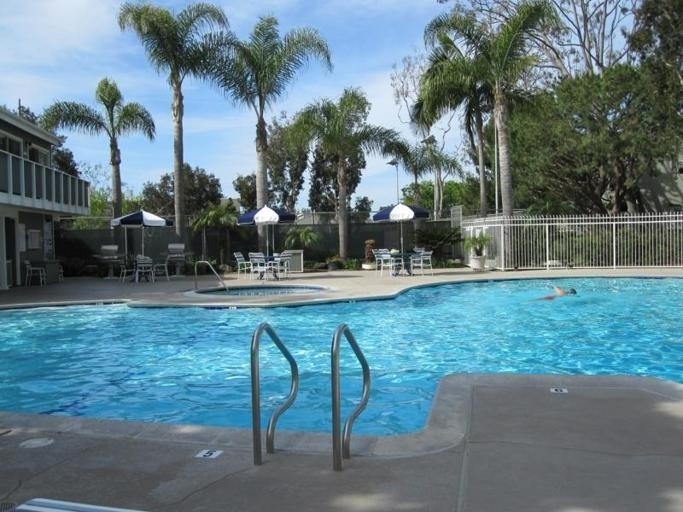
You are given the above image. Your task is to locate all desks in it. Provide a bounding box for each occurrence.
[389,251,415,275]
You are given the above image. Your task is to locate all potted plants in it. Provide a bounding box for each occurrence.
[360,237,376,271]
[463,232,491,272]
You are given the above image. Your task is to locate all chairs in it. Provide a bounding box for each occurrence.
[378,249,404,276]
[18,239,300,293]
[371,247,391,276]
[409,250,434,278]
[411,246,424,274]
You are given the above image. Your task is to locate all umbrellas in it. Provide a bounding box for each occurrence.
[372,202,429,277]
[111,209,173,282]
[237,204,297,281]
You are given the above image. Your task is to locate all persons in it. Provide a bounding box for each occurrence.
[534,285,577,299]
